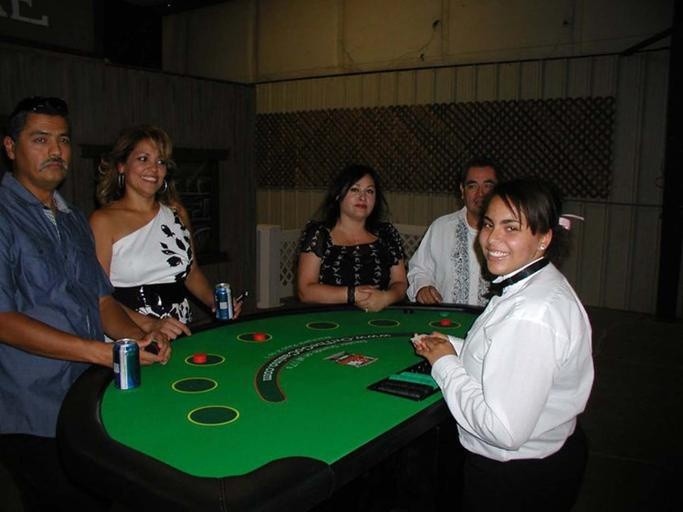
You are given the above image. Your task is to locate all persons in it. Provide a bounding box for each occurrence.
[412,176,595,512]
[87,122,243,341]
[296,165,409,313]
[407,159,500,307]
[0,97,173,454]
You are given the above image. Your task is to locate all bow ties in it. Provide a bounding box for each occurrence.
[484,283,505,298]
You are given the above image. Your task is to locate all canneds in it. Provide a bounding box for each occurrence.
[113,338,141,390]
[214,282,233,320]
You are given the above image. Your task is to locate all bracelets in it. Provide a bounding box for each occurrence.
[347,285,356,306]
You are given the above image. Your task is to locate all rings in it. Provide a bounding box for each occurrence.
[365,307,368,313]
